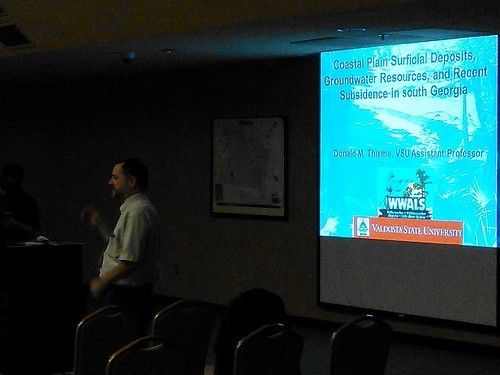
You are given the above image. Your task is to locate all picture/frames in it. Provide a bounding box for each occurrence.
[209,111,286,222]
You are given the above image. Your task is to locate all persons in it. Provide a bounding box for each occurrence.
[81,160,160,347]
[0,210,50,243]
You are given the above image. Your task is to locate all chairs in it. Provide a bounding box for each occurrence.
[72,288,392,375]
